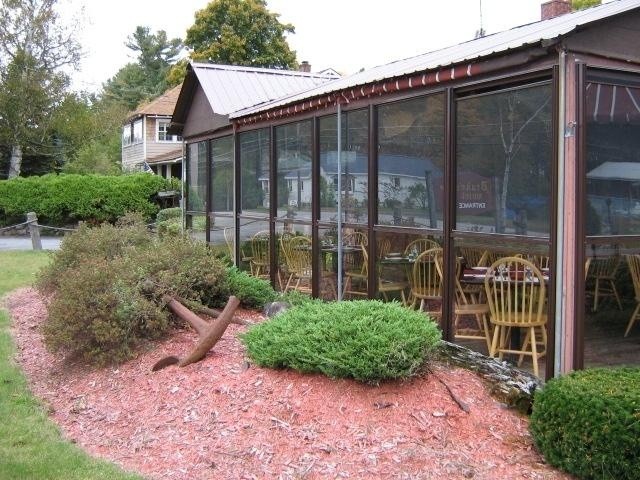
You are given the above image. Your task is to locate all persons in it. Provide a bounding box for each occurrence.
[512,206,528,234]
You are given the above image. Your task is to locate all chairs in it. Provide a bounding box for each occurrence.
[224,224,550,378]
[585,239,640,339]
